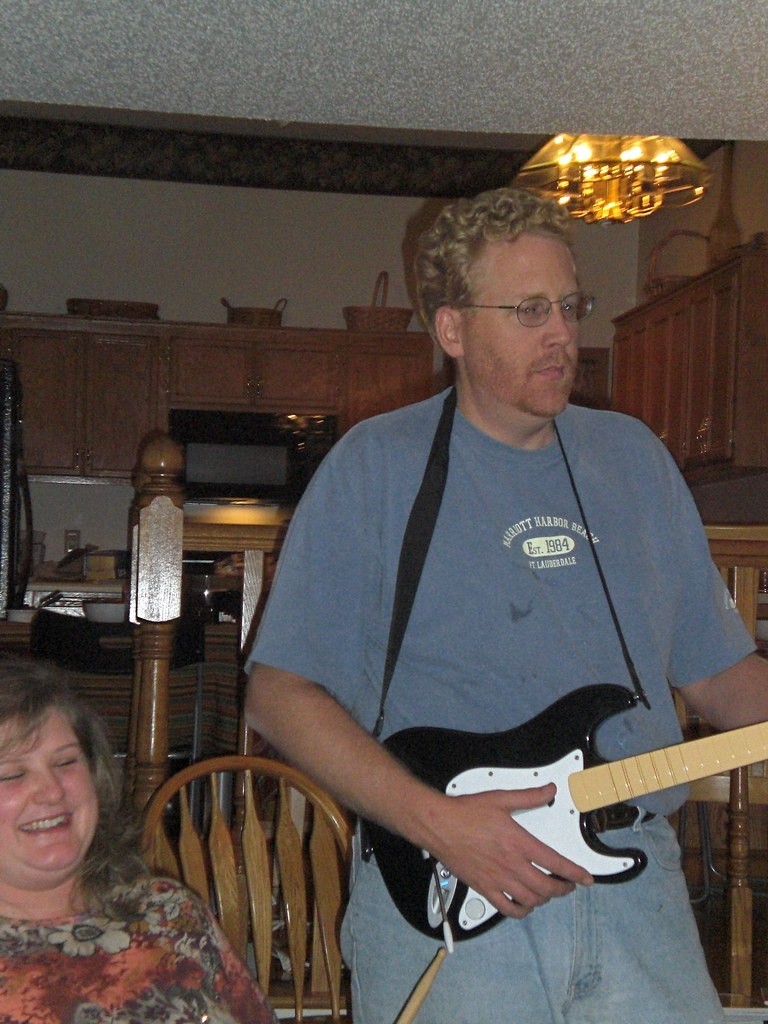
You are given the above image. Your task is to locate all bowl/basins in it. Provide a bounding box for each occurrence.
[82,599,128,624]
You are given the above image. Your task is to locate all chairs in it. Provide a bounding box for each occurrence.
[674,540,768,907]
[29,609,205,836]
[137,754,352,1024]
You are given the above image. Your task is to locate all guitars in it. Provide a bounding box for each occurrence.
[361,683,768,944]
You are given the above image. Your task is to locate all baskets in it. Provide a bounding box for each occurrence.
[214,297,288,328]
[66,297,158,321]
[642,229,717,298]
[341,270,415,334]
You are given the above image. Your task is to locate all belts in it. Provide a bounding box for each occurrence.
[594,804,657,828]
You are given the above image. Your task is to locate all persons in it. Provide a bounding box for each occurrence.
[245,188,767,1024]
[0,664,279,1024]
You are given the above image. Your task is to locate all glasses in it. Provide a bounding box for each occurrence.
[459,292,596,329]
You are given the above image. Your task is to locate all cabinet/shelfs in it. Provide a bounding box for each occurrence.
[611,252,768,488]
[164,319,349,415]
[0,310,165,487]
[338,329,611,436]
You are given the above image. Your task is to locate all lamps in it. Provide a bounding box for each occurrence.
[512,133,709,225]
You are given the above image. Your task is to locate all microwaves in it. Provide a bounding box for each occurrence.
[165,406,339,506]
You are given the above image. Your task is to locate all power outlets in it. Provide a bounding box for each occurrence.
[64,529,80,554]
